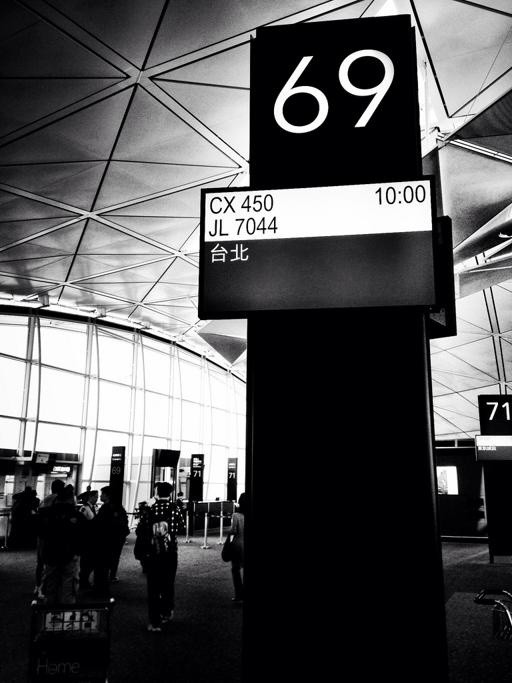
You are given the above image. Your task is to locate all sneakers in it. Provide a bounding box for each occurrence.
[147,610,174,632]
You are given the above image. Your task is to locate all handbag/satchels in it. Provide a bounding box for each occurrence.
[221,532,235,563]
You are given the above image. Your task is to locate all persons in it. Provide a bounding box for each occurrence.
[5,477,186,637]
[222,491,246,601]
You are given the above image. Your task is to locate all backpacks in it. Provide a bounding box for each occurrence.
[146,504,177,556]
